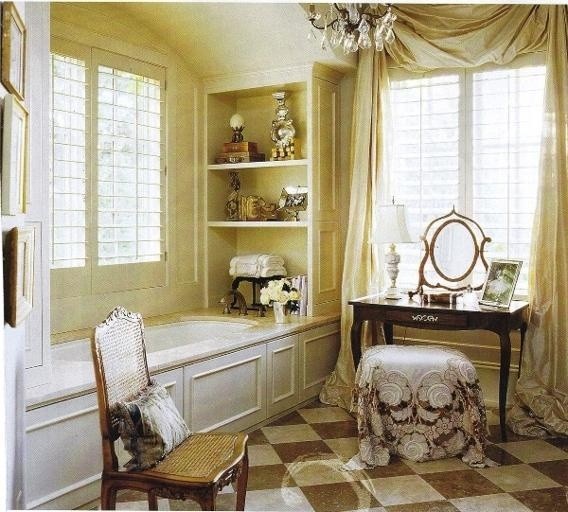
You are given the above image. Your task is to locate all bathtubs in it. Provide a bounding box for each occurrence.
[52,315,258,363]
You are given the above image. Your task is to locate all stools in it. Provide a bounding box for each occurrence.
[231,275,285,312]
[341,344,489,469]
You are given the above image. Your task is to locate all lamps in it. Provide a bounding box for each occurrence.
[367,195,415,300]
[305,3,398,56]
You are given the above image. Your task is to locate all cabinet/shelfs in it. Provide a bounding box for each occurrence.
[204,62,345,319]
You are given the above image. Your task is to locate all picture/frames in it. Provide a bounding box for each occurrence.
[278,187,308,211]
[1,1,28,101]
[478,258,523,310]
[4,226,36,328]
[1,94,30,216]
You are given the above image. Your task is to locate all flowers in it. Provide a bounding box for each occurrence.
[260,278,301,306]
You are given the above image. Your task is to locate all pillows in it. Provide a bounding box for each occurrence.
[110,380,193,471]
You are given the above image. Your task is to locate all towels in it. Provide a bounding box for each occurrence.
[229,268,288,277]
[229,255,284,268]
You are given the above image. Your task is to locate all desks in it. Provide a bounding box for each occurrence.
[348,292,530,444]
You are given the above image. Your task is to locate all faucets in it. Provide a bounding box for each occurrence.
[218,290,247,315]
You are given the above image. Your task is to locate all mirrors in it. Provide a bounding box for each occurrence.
[409,204,491,300]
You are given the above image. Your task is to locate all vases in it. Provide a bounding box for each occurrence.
[273,302,287,323]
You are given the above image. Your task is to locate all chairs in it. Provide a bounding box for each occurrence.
[91,306,249,511]
